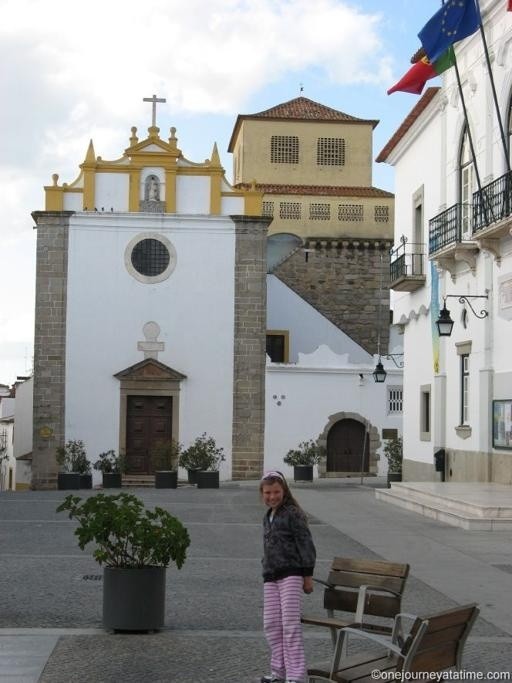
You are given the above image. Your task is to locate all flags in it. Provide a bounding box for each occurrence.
[386,44,457,95]
[417,0,483,65]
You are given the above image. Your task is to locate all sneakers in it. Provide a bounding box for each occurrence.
[262,675,282,683]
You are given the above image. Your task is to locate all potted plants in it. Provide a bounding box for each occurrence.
[383,437,403,488]
[56,492,191,630]
[74,452,92,489]
[149,440,184,488]
[180,445,210,484]
[55,440,85,490]
[92,450,129,488]
[195,433,226,489]
[283,440,327,481]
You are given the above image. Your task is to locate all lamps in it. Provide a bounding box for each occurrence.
[373,353,404,382]
[434,294,488,335]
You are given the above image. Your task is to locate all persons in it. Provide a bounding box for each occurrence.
[260,471,317,683]
[147,177,159,202]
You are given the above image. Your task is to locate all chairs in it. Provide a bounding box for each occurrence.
[307,602,480,683]
[301,556,410,648]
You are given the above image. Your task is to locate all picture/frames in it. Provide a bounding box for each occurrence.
[492,399,512,450]
